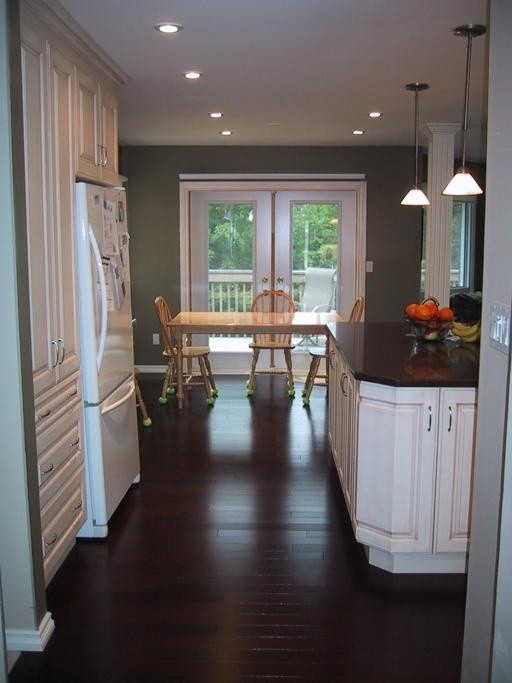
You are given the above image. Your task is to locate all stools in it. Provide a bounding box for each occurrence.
[134,367,152,426]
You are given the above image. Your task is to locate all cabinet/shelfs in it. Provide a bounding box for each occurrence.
[20,1,88,588]
[324,324,361,541]
[76,35,119,186]
[358,382,478,556]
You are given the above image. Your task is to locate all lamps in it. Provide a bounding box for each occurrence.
[401,81,432,206]
[440,25,486,196]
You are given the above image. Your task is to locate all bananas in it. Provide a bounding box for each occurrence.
[453,320,481,344]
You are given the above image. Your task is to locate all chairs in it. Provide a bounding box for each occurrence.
[289,267,336,345]
[154,296,218,405]
[302,297,364,406]
[245,290,297,396]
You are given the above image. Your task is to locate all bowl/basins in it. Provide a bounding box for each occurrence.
[406,319,451,343]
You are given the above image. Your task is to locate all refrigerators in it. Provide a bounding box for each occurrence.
[74,182,140,538]
